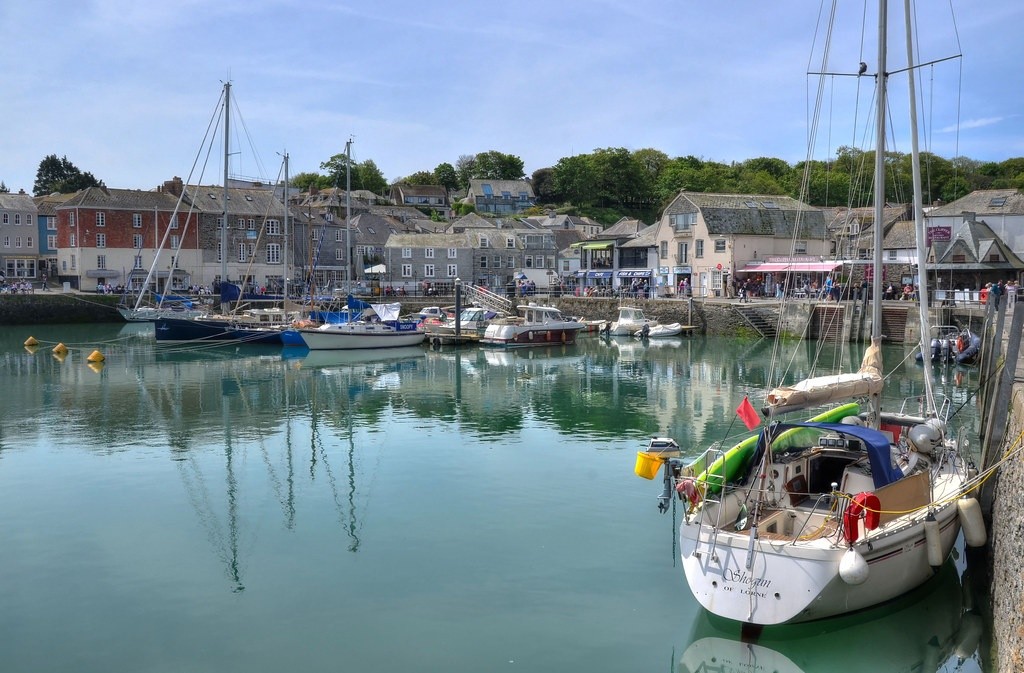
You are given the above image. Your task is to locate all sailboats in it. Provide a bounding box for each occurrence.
[114,75,702,348]
[636,0,1008,625]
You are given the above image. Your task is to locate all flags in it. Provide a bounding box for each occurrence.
[736,395,761,431]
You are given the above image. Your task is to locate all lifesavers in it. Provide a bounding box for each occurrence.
[843,495,881,542]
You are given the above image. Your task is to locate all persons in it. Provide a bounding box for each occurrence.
[2,274,50,293]
[98,282,127,295]
[518,278,689,299]
[250,282,432,297]
[738,277,1018,312]
[188,285,212,295]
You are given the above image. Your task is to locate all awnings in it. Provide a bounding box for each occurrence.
[738,262,842,272]
[571,241,614,249]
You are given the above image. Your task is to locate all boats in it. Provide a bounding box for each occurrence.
[914,330,981,368]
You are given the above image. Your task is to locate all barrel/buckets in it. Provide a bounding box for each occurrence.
[634,438,675,480]
